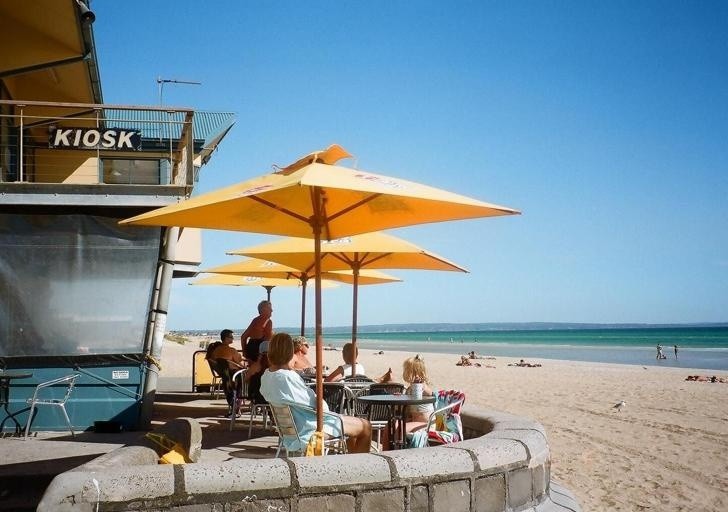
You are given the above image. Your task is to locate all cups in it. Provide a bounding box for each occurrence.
[411,382,427,397]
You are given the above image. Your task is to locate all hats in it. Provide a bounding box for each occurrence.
[259,340,270,355]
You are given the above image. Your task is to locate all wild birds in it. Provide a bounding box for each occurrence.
[612,400,627,411]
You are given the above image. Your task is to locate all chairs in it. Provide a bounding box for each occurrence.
[189,345,466,457]
[23,371,81,439]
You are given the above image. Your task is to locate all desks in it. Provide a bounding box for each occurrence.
[0,371,33,436]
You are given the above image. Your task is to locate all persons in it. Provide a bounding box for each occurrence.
[258,332,373,453]
[655,342,728,383]
[383,354,439,451]
[206,299,366,418]
[455,350,543,369]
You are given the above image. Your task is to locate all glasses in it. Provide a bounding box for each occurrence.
[228,336,234,339]
[301,344,308,347]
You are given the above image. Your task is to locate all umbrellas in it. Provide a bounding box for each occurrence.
[201,258,403,335]
[226,231,470,378]
[116,143,523,455]
[189,272,342,301]
